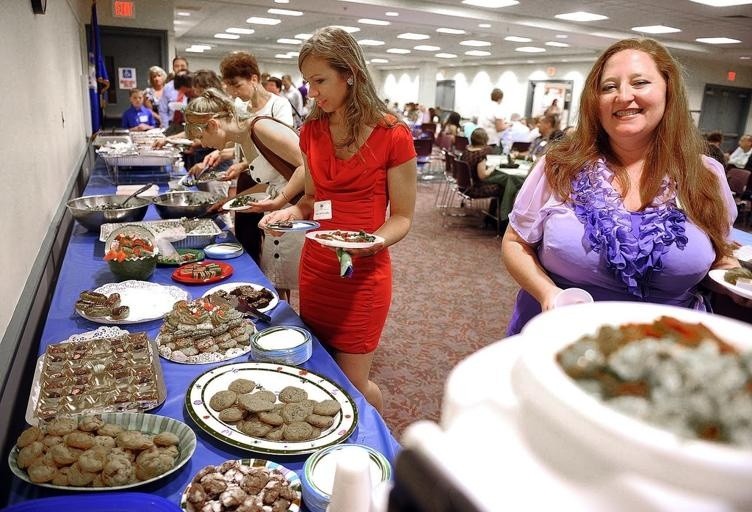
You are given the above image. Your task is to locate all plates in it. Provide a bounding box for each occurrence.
[23,331,168,427]
[104,224,156,255]
[300,444,392,512]
[179,457,302,512]
[221,193,271,211]
[156,248,206,267]
[250,324,313,365]
[9,412,198,491]
[183,361,359,456]
[265,219,320,233]
[154,321,260,365]
[75,279,193,326]
[171,260,234,286]
[204,243,243,259]
[200,281,280,315]
[302,231,385,248]
[707,269,752,301]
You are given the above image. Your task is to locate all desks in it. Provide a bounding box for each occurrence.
[9,122,402,512]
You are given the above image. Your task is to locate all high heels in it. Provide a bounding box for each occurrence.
[484,215,498,231]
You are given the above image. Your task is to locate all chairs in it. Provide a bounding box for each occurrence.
[411,123,533,236]
[727,169,751,228]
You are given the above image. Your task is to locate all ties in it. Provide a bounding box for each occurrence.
[174,92,184,125]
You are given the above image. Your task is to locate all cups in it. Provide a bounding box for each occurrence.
[553,287,594,309]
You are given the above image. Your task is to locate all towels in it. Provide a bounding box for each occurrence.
[337,250,352,278]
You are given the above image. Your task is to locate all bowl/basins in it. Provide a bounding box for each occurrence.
[63,188,220,232]
[106,248,158,281]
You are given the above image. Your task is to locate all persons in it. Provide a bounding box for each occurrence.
[118,52,314,303]
[378,86,581,232]
[498,38,739,346]
[260,24,420,413]
[701,132,751,174]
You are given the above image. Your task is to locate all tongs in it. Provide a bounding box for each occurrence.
[234,298,273,323]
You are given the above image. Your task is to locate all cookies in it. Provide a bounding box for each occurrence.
[158,300,255,363]
[209,379,340,441]
[16,414,180,487]
[186,459,297,512]
